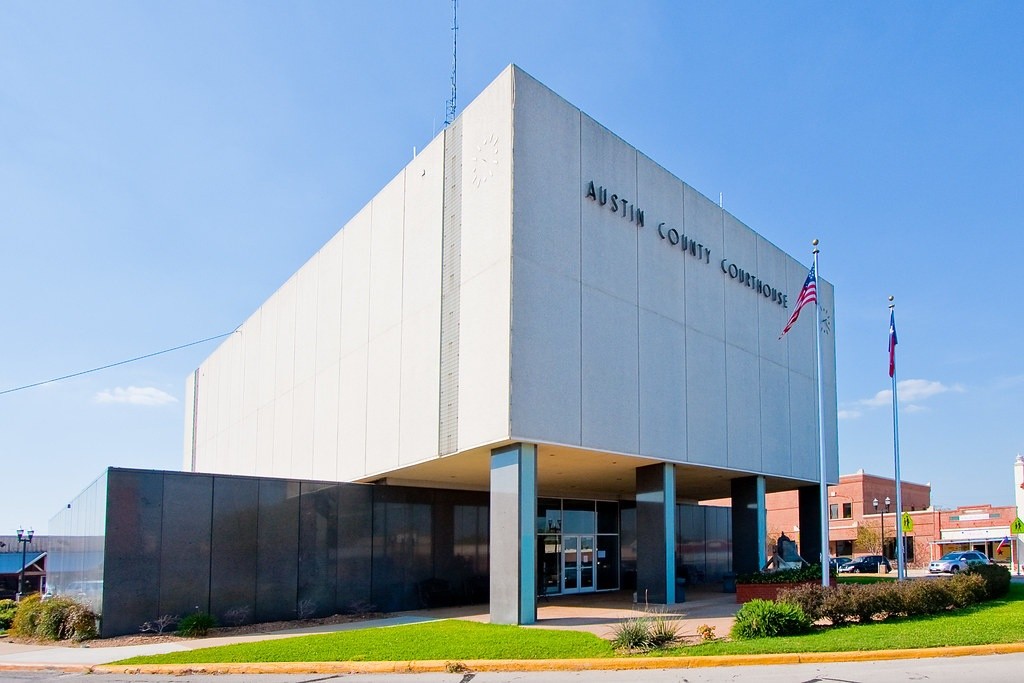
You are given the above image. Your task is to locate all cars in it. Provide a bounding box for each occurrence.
[929,549,990,574]
[840,555,893,575]
[829,557,855,571]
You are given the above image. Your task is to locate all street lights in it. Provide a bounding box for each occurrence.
[17,529,35,601]
[872,497,893,575]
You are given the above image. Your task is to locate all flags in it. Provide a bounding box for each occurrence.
[888,310,899,377]
[996,537,1009,554]
[777,260,818,339]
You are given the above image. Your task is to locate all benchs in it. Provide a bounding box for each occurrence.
[703,572,738,593]
[636,578,686,603]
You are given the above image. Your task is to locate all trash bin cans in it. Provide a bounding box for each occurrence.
[674,578,687,603]
[722,571,737,593]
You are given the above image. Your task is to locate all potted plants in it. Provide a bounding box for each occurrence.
[734,564,837,603]
